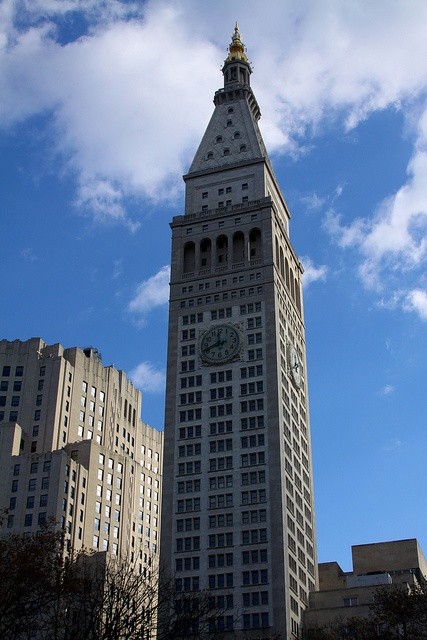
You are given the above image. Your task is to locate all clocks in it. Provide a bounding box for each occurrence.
[196,323,244,367]
[286,342,303,391]
